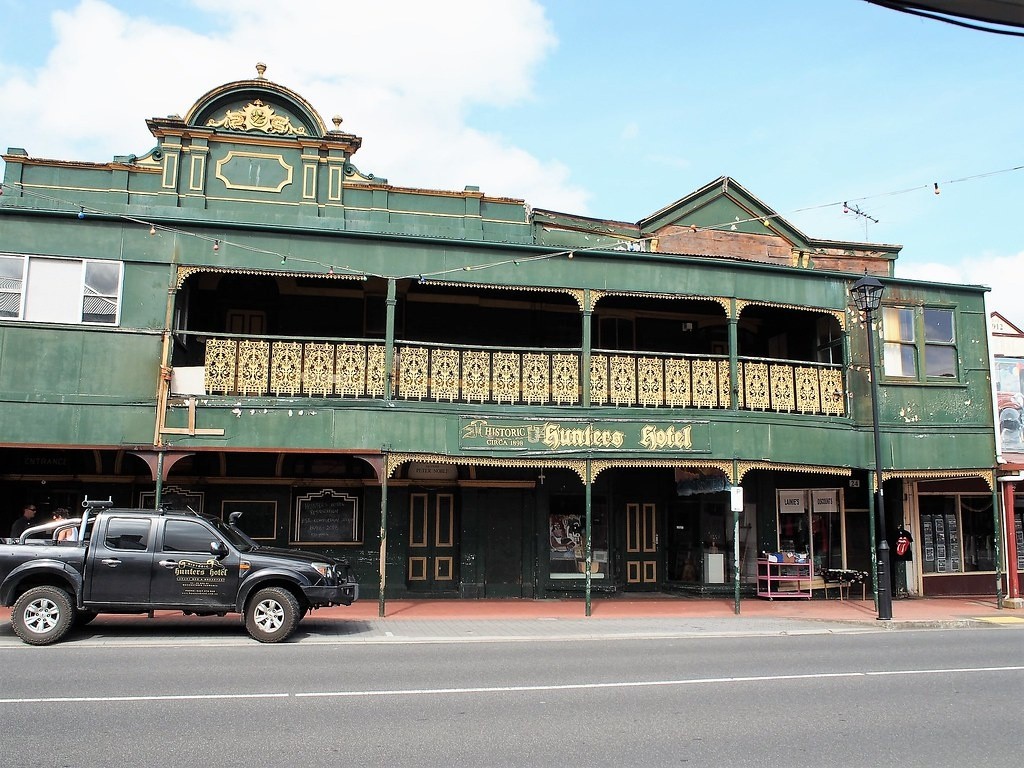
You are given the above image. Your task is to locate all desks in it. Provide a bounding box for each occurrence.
[824,570,866,601]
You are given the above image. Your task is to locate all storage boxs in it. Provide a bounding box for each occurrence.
[766,554,777,563]
[780,552,795,563]
[774,553,783,562]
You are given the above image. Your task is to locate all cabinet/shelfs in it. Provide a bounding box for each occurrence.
[755,558,813,601]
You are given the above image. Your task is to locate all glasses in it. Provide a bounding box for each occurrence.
[27,508,36,511]
[53,517,58,521]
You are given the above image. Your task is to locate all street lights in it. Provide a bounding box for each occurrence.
[849,266,897,620]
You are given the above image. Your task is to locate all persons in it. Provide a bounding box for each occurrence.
[49,507,78,542]
[8,503,39,544]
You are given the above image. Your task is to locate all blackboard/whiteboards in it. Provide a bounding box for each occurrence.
[288,486,364,546]
[221,500,277,540]
[131,483,212,513]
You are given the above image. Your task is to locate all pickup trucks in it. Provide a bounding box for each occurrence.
[0,495,360,645]
